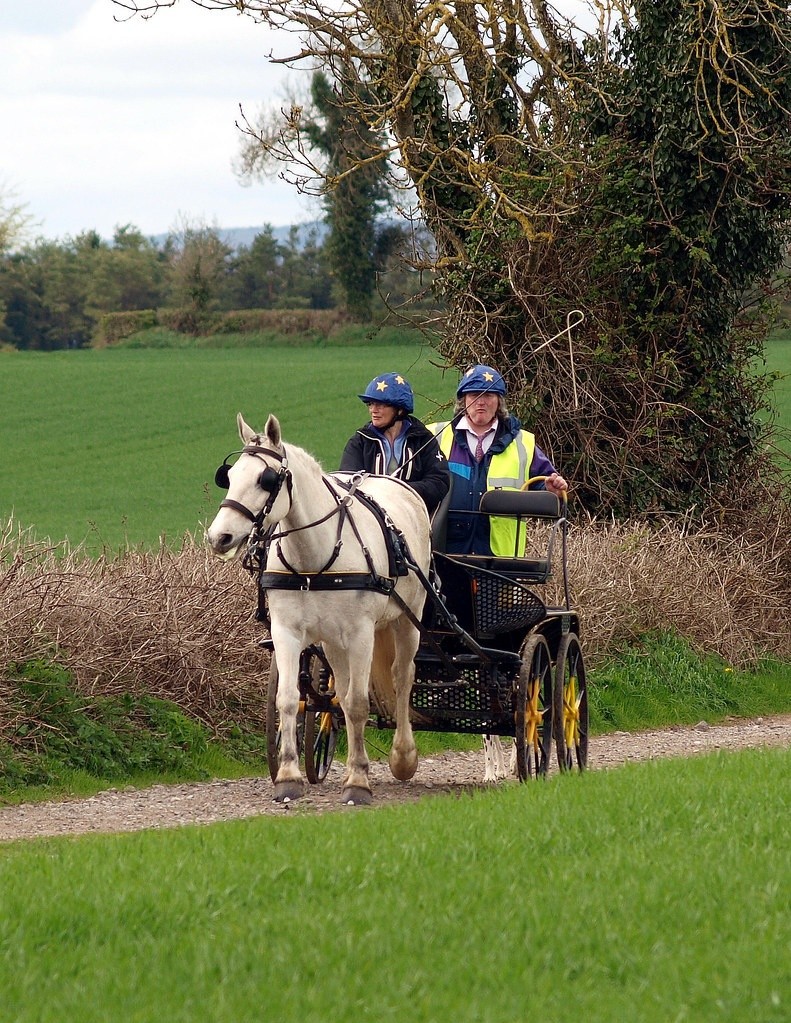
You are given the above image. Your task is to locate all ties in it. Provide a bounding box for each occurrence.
[473,430,493,462]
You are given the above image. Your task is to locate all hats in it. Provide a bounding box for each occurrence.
[359,372,413,414]
[456,365,506,398]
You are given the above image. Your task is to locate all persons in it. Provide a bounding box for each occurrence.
[424,365,569,617]
[338,373,451,505]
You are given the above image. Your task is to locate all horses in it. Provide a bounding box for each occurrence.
[206,411,432,807]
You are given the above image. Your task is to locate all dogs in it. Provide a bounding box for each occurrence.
[475,733,519,784]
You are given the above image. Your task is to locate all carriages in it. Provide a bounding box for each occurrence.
[207,412,589,806]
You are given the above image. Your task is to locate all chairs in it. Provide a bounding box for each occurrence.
[427,472,560,582]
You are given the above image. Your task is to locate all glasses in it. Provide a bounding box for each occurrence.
[365,402,392,409]
